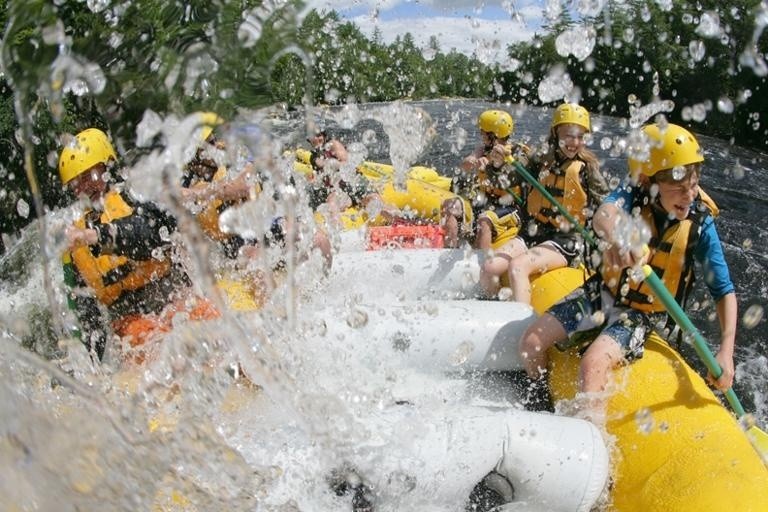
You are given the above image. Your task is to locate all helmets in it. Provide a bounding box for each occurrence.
[59,127,119,184]
[626,123,707,185]
[478,109,513,138]
[550,102,592,133]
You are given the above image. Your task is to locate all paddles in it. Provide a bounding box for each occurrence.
[642,265,768,456]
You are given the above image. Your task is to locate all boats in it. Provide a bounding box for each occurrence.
[1,149,767,512]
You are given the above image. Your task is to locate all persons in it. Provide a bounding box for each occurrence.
[516,122,737,451]
[440,108,532,252]
[479,101,610,305]
[55,103,379,400]
